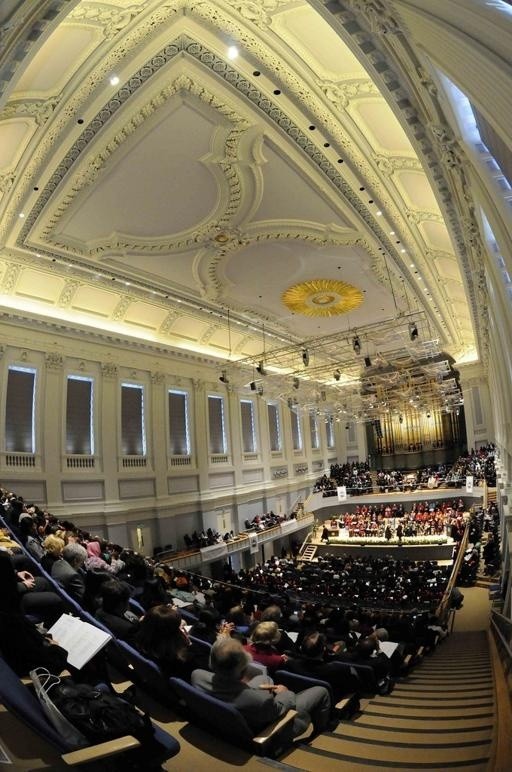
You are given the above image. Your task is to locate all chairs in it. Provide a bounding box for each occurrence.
[0,515,389,770]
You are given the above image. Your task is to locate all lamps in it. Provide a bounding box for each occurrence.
[212,253,466,431]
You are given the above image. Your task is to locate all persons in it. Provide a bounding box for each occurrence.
[0,430,511,772]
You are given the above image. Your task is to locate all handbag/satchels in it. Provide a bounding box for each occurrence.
[28,665,154,748]
